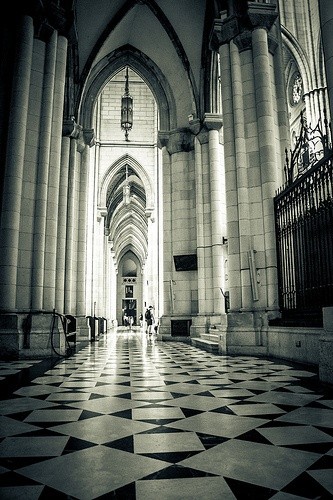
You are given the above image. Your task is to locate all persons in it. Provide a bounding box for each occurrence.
[123,312,134,328]
[139,312,144,327]
[145,306,155,335]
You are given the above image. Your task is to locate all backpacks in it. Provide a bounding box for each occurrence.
[145,309,151,319]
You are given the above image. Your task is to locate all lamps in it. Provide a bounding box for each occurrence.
[121,165,130,208]
[120,66,134,142]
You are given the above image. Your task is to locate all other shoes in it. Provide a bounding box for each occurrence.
[146,330,147,334]
[149,333,152,335]
